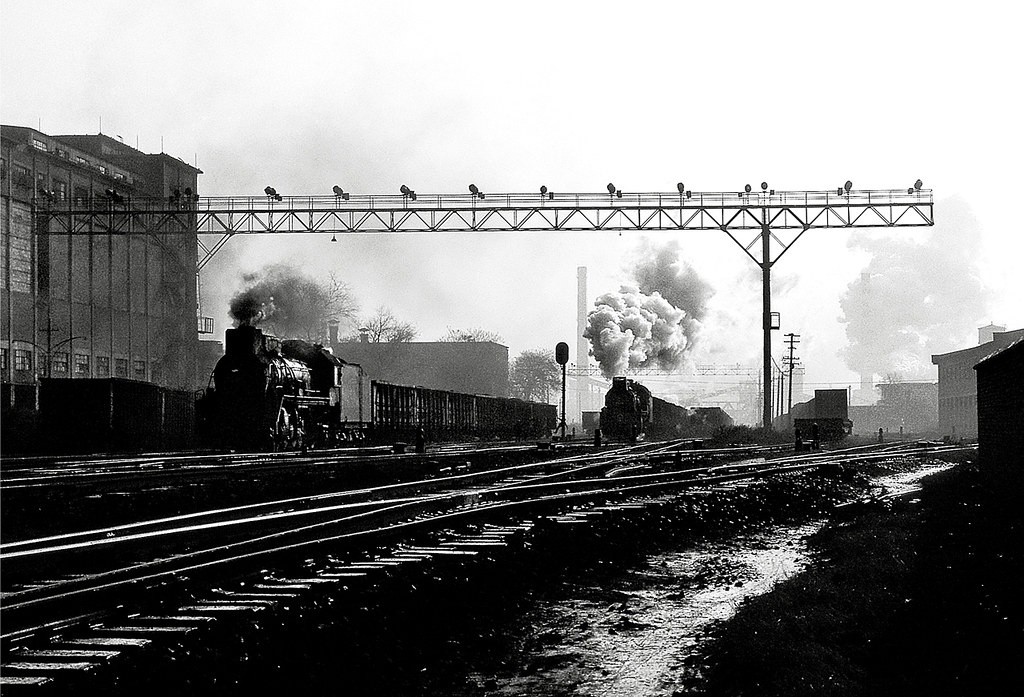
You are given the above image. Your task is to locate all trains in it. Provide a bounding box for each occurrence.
[187,309,559,454]
[597,376,736,443]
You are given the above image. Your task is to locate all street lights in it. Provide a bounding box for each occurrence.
[10,314,90,377]
